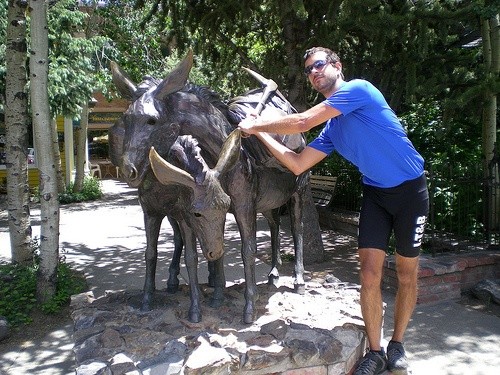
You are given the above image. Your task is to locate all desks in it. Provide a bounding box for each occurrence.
[100,161,115,179]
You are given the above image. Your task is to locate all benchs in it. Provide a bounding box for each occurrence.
[309,176,338,232]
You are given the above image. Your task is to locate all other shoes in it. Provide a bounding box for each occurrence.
[387,340,409,372]
[350,347,389,374]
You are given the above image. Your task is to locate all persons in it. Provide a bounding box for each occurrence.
[237,47,430,374]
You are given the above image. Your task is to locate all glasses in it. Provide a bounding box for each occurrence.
[306,60,333,72]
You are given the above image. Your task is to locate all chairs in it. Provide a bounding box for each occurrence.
[88,161,102,179]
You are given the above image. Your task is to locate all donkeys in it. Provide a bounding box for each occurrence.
[109,49,310,324]
